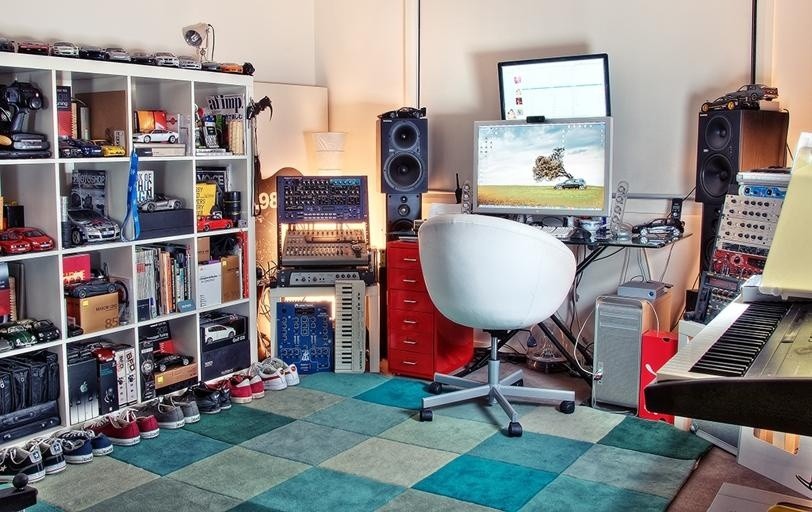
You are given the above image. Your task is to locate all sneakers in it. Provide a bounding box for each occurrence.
[133,398,184,429]
[161,394,202,424]
[169,384,222,415]
[118,407,160,439]
[260,358,299,386]
[197,380,232,409]
[79,415,141,447]
[51,429,114,457]
[1,446,44,484]
[63,439,93,464]
[34,438,68,474]
[235,373,266,400]
[241,362,287,390]
[207,376,253,404]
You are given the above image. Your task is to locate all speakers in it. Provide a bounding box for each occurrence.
[697,202,723,287]
[460,179,471,215]
[381,116,429,193]
[387,193,421,241]
[611,180,634,237]
[695,109,791,202]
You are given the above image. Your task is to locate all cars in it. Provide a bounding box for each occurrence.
[554,178,584,189]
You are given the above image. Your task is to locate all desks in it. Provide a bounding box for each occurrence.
[405,230,689,377]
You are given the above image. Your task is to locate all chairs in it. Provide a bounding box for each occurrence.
[414,213,578,438]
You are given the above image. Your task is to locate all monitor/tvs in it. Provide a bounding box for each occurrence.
[471,116,616,227]
[497,53,612,120]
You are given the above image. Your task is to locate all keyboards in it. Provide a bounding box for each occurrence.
[529,224,574,239]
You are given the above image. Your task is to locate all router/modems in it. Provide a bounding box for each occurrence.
[590,293,672,417]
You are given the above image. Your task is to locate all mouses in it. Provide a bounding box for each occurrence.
[573,226,592,241]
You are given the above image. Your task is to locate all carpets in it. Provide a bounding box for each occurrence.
[0,368,716,512]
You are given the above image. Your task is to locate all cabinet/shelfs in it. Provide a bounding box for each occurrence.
[387,240,473,380]
[0,48,259,458]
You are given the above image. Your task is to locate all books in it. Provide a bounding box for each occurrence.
[9,276,18,322]
[133,143,186,149]
[135,243,195,320]
[61,196,72,247]
[164,112,181,144]
[196,121,245,156]
[143,148,186,157]
[9,262,26,320]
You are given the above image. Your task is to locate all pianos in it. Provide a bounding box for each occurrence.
[334,279,365,374]
[644,295,811,438]
